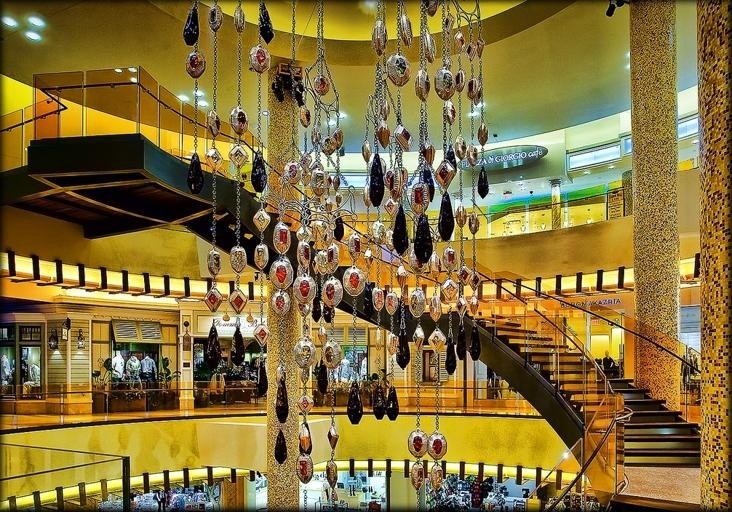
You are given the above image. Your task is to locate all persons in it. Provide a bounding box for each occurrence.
[333,357,367,381]
[112,354,158,389]
[156,490,167,512]
